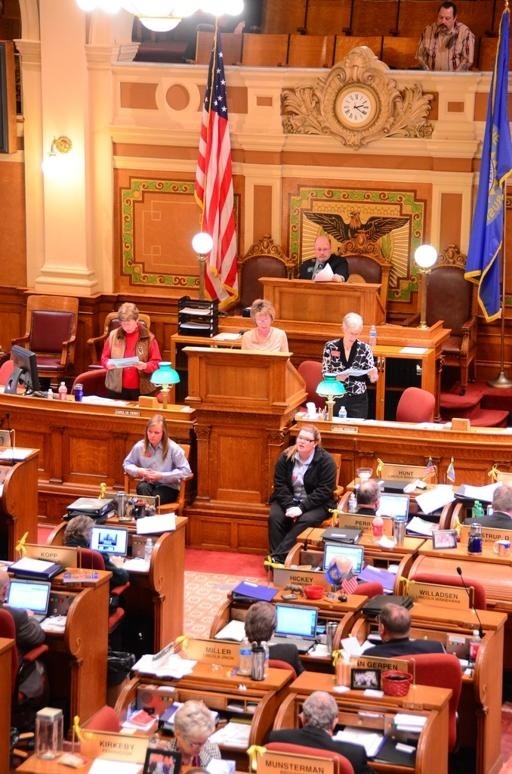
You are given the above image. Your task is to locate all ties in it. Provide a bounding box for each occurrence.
[312,265,322,281]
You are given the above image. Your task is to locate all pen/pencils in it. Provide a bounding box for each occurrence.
[333,371,349,378]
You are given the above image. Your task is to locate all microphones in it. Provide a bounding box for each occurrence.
[5,413,16,466]
[231,330,244,348]
[457,567,483,639]
[289,259,315,280]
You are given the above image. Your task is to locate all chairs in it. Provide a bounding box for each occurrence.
[87,312,151,370]
[0,360,16,384]
[70,369,108,397]
[10,294,79,378]
[334,232,392,324]
[399,243,479,395]
[218,232,298,315]
[296,360,325,412]
[394,387,436,425]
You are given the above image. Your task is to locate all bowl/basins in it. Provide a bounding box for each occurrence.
[303,585,324,599]
[382,671,413,697]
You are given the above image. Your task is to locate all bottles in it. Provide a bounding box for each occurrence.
[325,621,338,654]
[34,706,66,761]
[369,325,377,348]
[371,516,385,542]
[348,492,358,514]
[465,523,485,553]
[45,381,86,403]
[144,538,155,564]
[469,629,482,664]
[240,637,270,681]
[338,405,348,419]
[116,493,160,521]
[471,500,484,519]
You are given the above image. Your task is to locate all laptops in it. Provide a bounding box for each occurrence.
[323,543,364,581]
[7,580,51,623]
[375,493,410,526]
[90,526,128,557]
[268,603,318,654]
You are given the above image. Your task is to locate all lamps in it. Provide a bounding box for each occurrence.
[49,137,72,155]
[191,232,213,300]
[414,245,437,329]
[316,373,347,421]
[150,362,181,409]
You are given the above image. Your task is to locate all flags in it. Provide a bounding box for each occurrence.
[190,27,240,316]
[460,5,511,322]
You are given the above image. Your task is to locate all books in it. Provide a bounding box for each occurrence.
[211,332,241,341]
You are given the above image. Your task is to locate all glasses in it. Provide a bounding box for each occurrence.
[181,734,209,749]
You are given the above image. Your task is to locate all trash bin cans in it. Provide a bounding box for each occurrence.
[106,651,135,709]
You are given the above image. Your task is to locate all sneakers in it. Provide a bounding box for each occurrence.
[263,555,284,569]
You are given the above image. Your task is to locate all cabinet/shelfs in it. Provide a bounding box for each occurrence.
[367,345,435,421]
[170,332,243,405]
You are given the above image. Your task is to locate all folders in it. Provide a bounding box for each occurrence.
[453,483,478,499]
[232,581,278,602]
[320,527,362,544]
[355,564,396,594]
[158,701,219,731]
[406,516,439,536]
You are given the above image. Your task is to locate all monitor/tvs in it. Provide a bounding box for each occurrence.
[4,345,38,394]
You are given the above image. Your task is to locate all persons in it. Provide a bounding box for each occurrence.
[320,310,379,418]
[465,485,512,530]
[165,699,220,766]
[417,2,475,68]
[263,423,339,572]
[363,603,448,658]
[244,602,304,676]
[0,569,52,727]
[123,414,193,505]
[294,233,353,282]
[267,692,368,774]
[356,483,380,516]
[66,515,149,652]
[101,301,164,400]
[241,298,291,352]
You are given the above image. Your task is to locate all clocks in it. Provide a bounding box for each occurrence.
[333,82,381,133]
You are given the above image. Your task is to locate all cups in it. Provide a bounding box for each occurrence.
[493,540,511,558]
[393,518,407,545]
[356,467,373,485]
